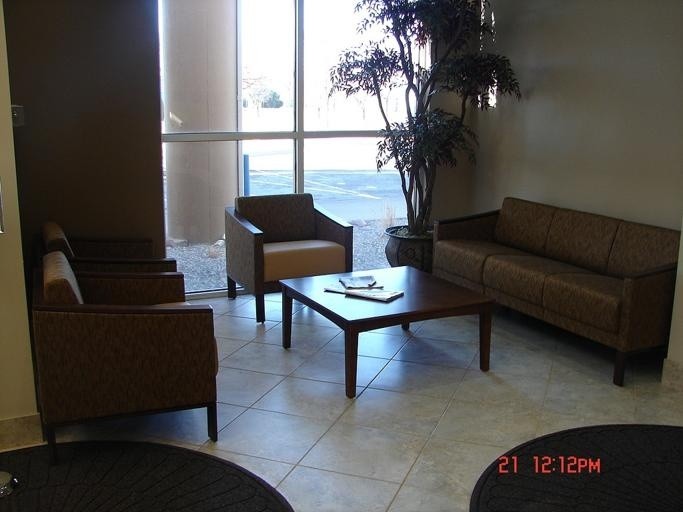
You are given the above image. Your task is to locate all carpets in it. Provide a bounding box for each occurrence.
[470,424,683,510]
[1,439,294,512]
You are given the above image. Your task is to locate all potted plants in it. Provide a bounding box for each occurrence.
[328,0,521,274]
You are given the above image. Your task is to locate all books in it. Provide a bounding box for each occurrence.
[325,274,404,303]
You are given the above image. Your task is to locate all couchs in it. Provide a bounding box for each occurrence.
[431,196,681,386]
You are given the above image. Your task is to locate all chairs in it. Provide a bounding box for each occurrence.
[28,222,220,454]
[224,194,353,323]
